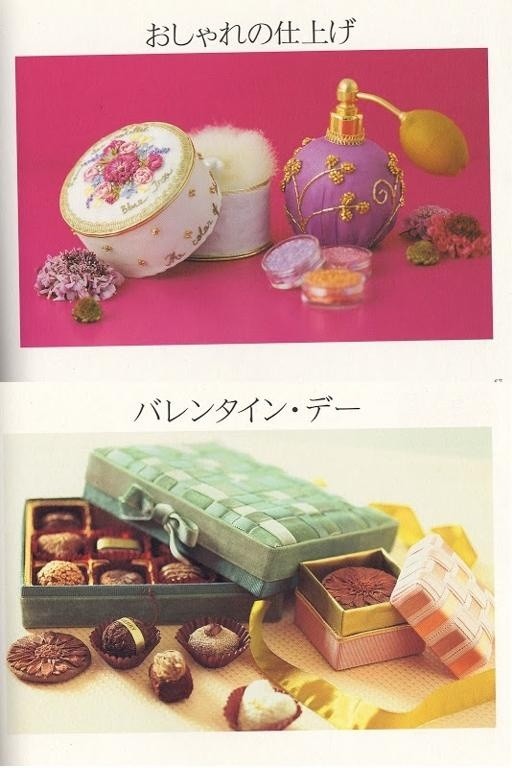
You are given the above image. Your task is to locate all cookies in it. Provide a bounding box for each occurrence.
[5,632,89,684]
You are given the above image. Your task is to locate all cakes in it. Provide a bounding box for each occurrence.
[97,570,147,584]
[150,554,215,583]
[224,679,301,731]
[177,617,250,668]
[90,524,146,555]
[148,648,193,703]
[36,559,82,585]
[323,567,397,607]
[32,527,89,559]
[35,505,84,529]
[90,615,162,668]
